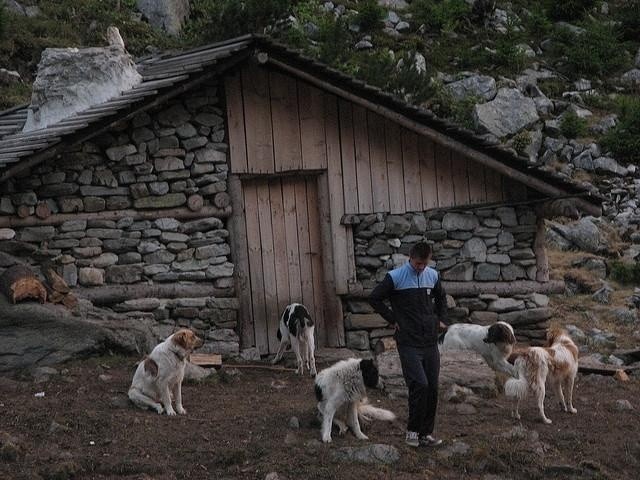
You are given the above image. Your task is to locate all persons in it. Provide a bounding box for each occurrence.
[367,243,449,447]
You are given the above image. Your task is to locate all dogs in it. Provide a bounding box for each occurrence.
[438,321,519,381]
[125,328,204,417]
[312,357,396,444]
[269,302,319,378]
[504,325,580,424]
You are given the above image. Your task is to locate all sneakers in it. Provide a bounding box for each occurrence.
[420,436,442,447]
[406,431,419,446]
